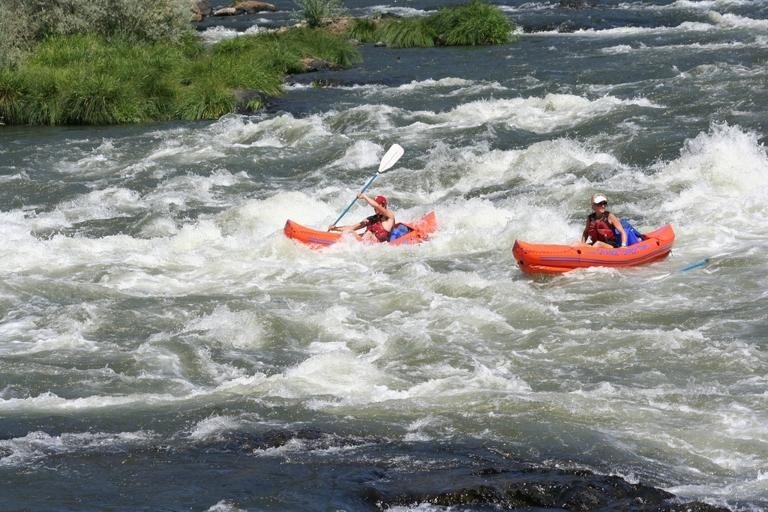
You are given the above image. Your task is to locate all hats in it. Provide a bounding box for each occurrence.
[591,192,609,205]
[373,196,387,205]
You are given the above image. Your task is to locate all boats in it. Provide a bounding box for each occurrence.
[512,221,674,283]
[280,208,440,257]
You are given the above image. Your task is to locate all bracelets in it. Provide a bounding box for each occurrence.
[621,242,627,247]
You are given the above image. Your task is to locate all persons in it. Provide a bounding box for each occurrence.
[330,192,395,244]
[581,193,628,248]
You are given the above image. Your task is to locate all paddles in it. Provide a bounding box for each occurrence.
[329,142,404,230]
[649,253,731,282]
